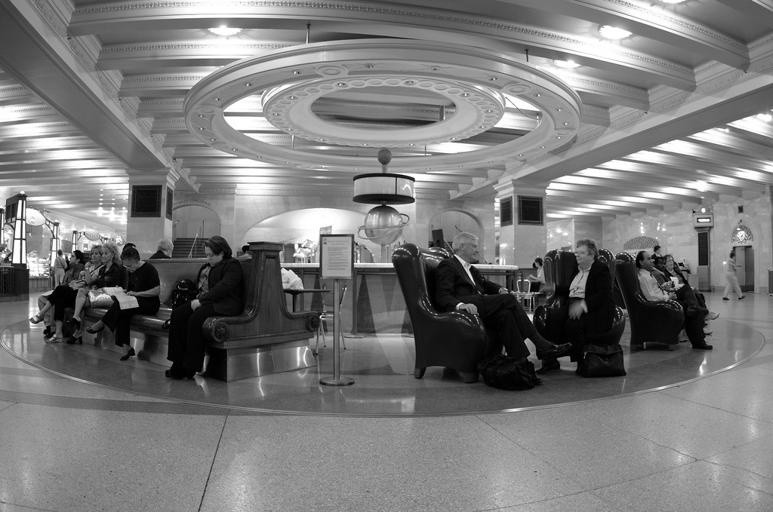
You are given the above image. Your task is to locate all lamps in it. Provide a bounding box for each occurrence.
[352,164,416,204]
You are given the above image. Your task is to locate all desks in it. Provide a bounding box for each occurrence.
[511,292,543,313]
[283,288,330,333]
[0,265,14,297]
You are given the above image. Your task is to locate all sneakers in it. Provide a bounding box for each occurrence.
[704,311,720,321]
[703,327,712,335]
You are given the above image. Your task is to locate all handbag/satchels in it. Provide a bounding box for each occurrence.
[69,278,87,291]
[575,345,627,379]
[481,356,538,392]
[88,289,114,308]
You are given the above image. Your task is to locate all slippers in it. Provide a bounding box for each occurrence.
[30,313,45,324]
[46,334,63,343]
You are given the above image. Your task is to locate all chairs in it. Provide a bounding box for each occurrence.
[314,284,350,355]
[517,280,532,312]
[615,252,684,353]
[653,258,706,303]
[393,242,504,385]
[534,246,628,363]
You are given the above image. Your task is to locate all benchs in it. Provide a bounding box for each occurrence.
[38,251,318,346]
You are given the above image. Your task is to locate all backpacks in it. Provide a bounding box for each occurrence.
[163,279,197,310]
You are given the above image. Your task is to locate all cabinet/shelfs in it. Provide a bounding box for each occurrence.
[13,269,29,295]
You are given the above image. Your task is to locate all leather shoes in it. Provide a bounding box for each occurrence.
[540,342,572,358]
[165,361,186,378]
[175,365,196,381]
[535,362,561,374]
[692,344,713,351]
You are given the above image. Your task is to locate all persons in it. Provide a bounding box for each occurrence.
[650,245,664,267]
[165,235,246,378]
[636,250,714,350]
[534,239,614,375]
[28,238,171,360]
[722,252,746,301]
[529,257,546,291]
[431,232,573,386]
[662,253,720,335]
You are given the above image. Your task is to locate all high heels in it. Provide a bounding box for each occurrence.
[66,335,83,345]
[120,348,135,361]
[65,317,83,330]
[86,324,105,334]
